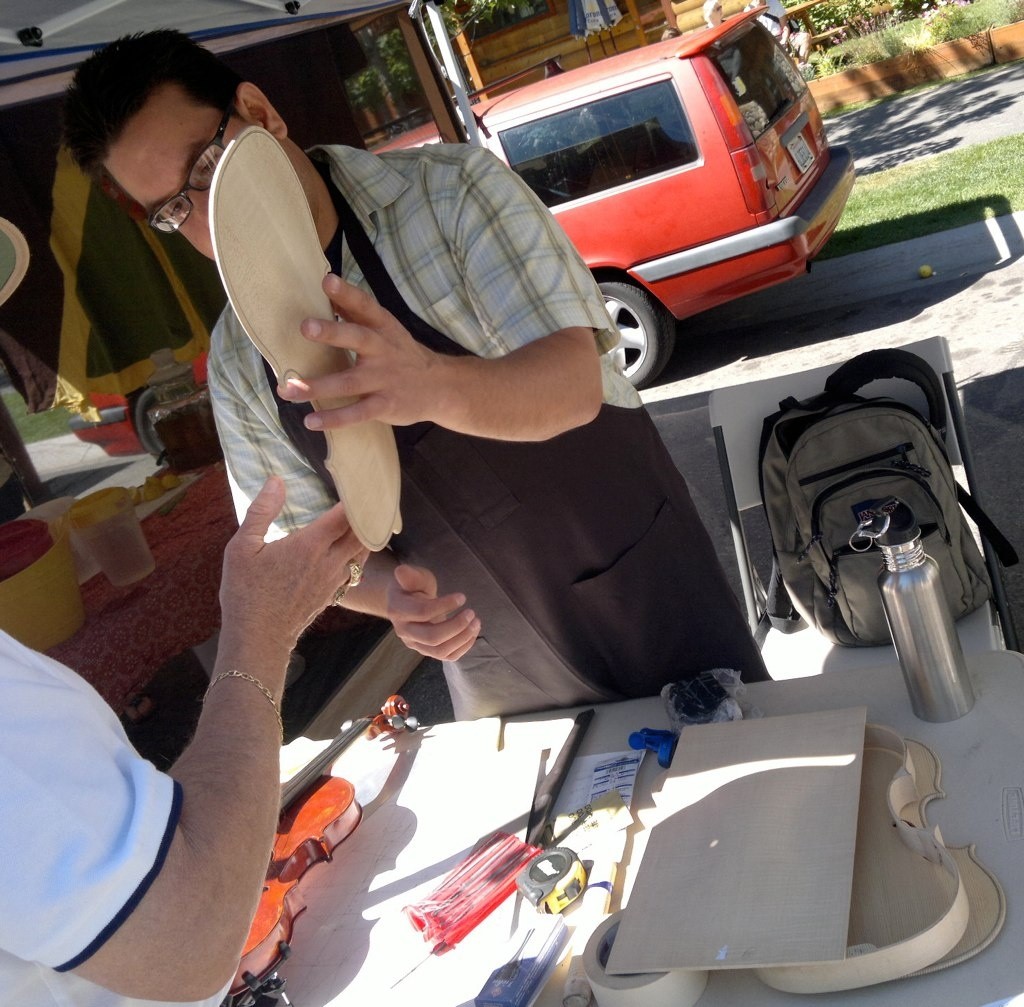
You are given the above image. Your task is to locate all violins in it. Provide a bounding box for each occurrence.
[224,694,420,1001]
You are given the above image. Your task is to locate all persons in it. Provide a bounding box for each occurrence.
[744,0,811,70]
[661,27,680,41]
[544,57,564,79]
[0,474,371,1007]
[62,28,772,721]
[702,0,746,79]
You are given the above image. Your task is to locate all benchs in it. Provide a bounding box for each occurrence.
[810,26,849,44]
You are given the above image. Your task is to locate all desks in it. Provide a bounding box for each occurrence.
[275,650,1024,1007]
[14,432,379,716]
[785,0,827,53]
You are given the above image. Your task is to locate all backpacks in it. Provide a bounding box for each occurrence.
[753,347,1021,648]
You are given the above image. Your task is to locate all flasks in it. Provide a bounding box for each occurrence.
[858,496,974,724]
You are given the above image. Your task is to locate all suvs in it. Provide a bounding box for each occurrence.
[71,8,856,474]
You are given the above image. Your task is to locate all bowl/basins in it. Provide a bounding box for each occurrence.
[0,531,83,652]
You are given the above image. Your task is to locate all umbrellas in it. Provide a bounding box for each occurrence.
[567,0,623,65]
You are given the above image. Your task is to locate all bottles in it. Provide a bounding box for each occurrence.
[148,348,196,404]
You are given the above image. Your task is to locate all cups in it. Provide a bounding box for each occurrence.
[68,488,154,585]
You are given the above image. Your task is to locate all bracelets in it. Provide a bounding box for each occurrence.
[203,670,283,741]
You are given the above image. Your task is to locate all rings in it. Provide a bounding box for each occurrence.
[331,586,345,606]
[345,559,365,587]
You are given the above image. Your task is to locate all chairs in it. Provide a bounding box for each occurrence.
[709,336,1019,681]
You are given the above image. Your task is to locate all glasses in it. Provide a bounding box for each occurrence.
[145,104,233,234]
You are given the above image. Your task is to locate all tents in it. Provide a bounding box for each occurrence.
[0,0,483,154]
